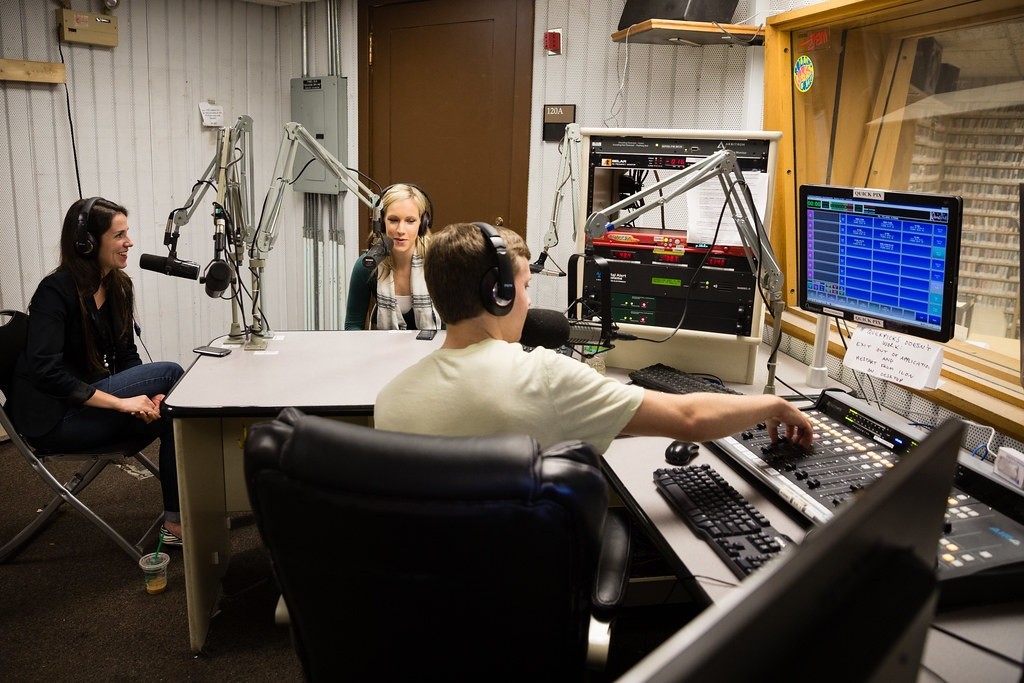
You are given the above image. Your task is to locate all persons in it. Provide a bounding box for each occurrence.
[345,184,446,331]
[21,197,185,545]
[374,222,813,450]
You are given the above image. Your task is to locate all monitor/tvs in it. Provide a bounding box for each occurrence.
[799,183,963,344]
[613,417,968,683]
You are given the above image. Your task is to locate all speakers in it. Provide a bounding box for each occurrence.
[912,37,960,106]
[617,0,739,30]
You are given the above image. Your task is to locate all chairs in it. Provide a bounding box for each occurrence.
[613,415,1024,683]
[0,310,166,563]
[244,410,630,683]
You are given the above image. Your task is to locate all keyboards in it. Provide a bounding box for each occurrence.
[652,464,797,582]
[628,363,743,394]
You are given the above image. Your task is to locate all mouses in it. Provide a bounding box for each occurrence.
[665,441,699,465]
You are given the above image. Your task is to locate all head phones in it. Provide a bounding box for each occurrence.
[377,183,434,237]
[471,222,517,317]
[73,197,103,260]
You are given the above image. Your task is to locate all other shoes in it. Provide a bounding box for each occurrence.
[160,525,183,545]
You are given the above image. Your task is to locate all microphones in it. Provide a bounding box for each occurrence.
[139,254,201,281]
[518,309,638,350]
[529,263,567,276]
[204,263,231,298]
[363,236,395,270]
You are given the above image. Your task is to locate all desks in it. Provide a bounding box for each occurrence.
[158,333,1024,683]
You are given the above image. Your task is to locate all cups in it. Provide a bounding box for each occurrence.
[139,552,170,594]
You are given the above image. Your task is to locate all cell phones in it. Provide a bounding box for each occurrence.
[416,330,437,340]
[192,346,232,357]
